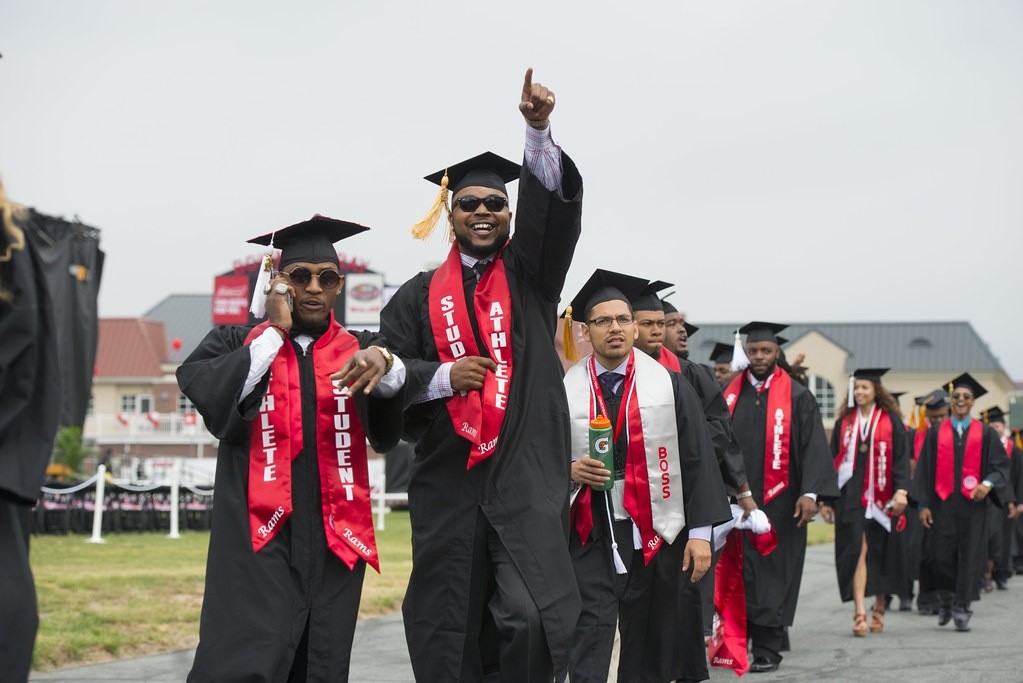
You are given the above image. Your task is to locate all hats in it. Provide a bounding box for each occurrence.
[633,280,676,312]
[412,150,522,244]
[979,405,1011,424]
[586,314,634,327]
[891,389,950,431]
[1009,427,1023,450]
[660,291,678,314]
[685,321,791,371]
[941,373,988,399]
[247,214,370,320]
[848,367,890,408]
[560,260,650,362]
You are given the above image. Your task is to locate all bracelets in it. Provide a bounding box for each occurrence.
[268,323,291,338]
[897,489,907,495]
[983,480,992,486]
[736,491,752,500]
[525,119,548,127]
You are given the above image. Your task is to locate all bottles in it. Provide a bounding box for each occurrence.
[589,415,614,491]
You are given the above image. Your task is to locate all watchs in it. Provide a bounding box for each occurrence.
[368,345,393,374]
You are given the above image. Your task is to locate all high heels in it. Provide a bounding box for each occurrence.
[868,608,885,633]
[853,614,867,636]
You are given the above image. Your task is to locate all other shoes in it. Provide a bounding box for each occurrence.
[900,593,914,610]
[955,616,970,631]
[937,592,953,625]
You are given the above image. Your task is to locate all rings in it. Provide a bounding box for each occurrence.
[547,96,555,104]
[263,281,272,295]
[275,283,288,294]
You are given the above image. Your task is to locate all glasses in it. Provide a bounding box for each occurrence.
[278,267,342,289]
[450,192,509,212]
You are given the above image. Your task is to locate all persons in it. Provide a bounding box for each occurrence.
[379,67,584,683]
[174,215,407,683]
[0,178,62,683]
[560,268,1023,683]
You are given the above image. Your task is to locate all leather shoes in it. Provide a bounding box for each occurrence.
[749,657,778,672]
